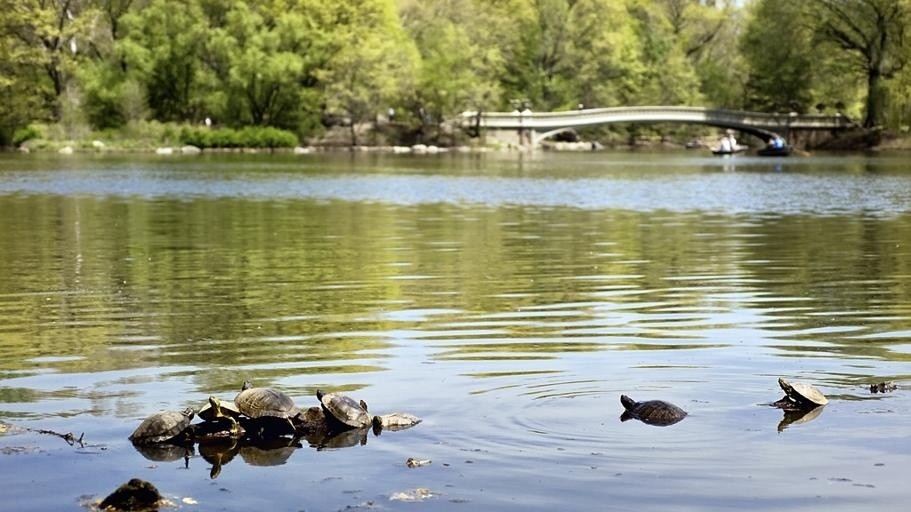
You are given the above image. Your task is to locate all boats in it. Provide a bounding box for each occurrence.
[810,124,884,152]
[757,144,792,155]
[686,141,706,150]
[713,145,748,157]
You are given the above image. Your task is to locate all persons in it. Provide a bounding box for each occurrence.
[728,131,739,151]
[723,153,730,171]
[775,156,783,172]
[769,132,785,150]
[715,134,731,152]
[731,154,737,170]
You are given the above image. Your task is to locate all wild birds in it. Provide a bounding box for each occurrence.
[405,457,432,468]
